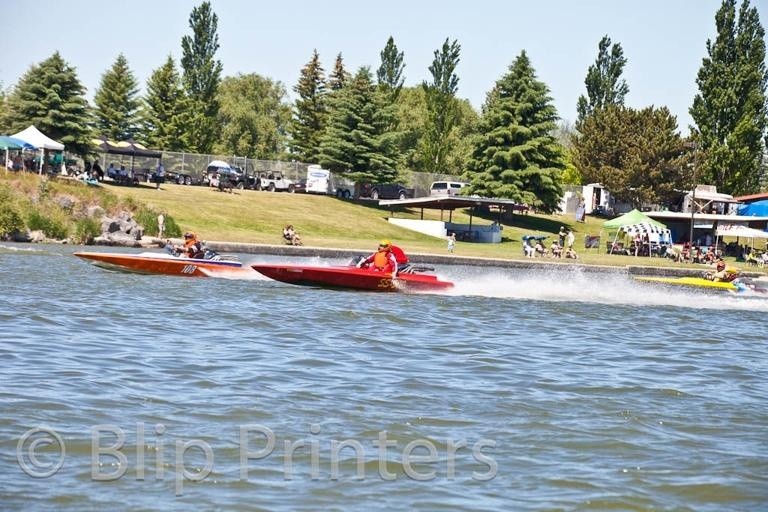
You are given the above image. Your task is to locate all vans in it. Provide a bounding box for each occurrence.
[428,180,470,197]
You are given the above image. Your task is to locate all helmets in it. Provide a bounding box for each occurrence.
[379,240,392,251]
[184,231,195,243]
[717,262,725,271]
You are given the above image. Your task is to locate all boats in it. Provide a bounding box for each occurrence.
[249,258,451,292]
[73,244,254,279]
[628,271,742,295]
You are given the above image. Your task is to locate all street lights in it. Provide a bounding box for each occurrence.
[681,140,697,264]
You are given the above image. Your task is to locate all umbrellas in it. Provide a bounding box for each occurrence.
[207,160,230,173]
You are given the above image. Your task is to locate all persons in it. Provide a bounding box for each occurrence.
[661,241,768,266]
[175,232,202,258]
[93,158,165,191]
[210,176,226,193]
[707,262,727,280]
[286,224,303,246]
[447,233,456,254]
[359,240,398,277]
[523,227,578,259]
